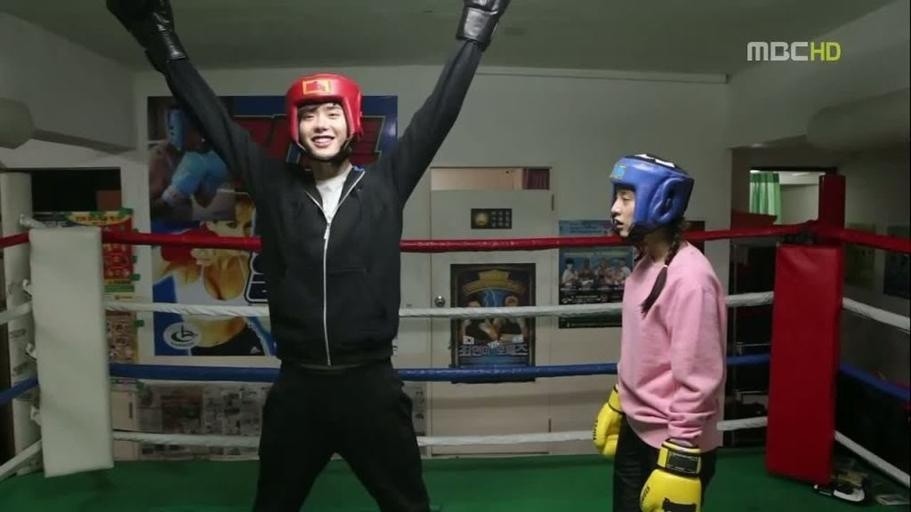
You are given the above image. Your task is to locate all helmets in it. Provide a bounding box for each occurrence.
[164,107,188,150]
[285,75,363,145]
[608,157,694,242]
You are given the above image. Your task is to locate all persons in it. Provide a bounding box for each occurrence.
[148,97,240,227]
[457,299,499,346]
[490,292,530,342]
[149,189,265,358]
[562,255,632,290]
[588,150,734,512]
[102,0,513,512]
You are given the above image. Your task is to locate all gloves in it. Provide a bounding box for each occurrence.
[206,151,238,180]
[454,0,510,48]
[594,384,623,457]
[161,151,205,209]
[160,230,220,267]
[640,441,701,512]
[105,0,186,70]
